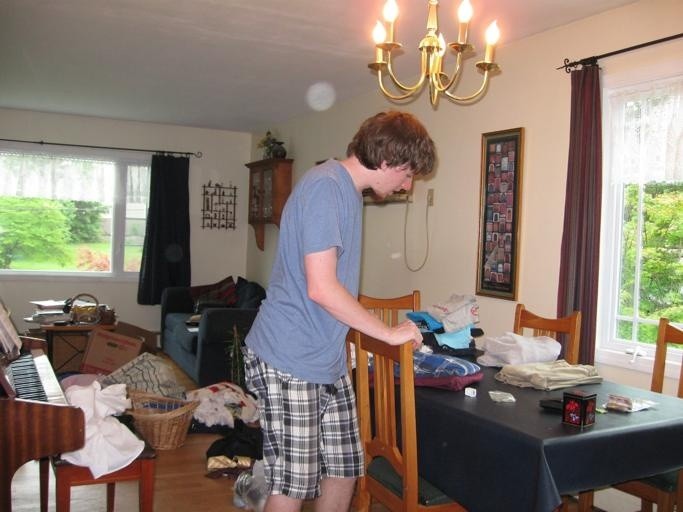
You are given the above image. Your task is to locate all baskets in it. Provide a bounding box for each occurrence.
[112,388,201,451]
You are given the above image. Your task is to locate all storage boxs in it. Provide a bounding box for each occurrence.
[78,327,145,376]
[563,388,597,430]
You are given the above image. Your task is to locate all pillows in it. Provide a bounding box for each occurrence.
[188,275,265,315]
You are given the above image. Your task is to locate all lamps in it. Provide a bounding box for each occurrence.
[365,0,501,110]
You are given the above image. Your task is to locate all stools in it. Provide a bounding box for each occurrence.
[48,430,158,512]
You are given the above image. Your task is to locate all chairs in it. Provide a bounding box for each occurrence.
[355,291,421,332]
[512,301,582,366]
[351,322,471,512]
[573,317,681,512]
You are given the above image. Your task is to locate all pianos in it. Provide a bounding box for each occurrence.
[0,336,84,511]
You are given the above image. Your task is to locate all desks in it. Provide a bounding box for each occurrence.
[39,323,114,369]
[349,343,683,512]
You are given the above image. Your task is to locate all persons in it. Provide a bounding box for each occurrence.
[243,111,436,512]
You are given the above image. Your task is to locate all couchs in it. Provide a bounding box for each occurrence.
[159,278,264,387]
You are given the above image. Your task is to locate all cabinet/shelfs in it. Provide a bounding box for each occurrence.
[242,157,292,250]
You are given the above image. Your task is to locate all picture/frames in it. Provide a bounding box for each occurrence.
[473,126,526,304]
